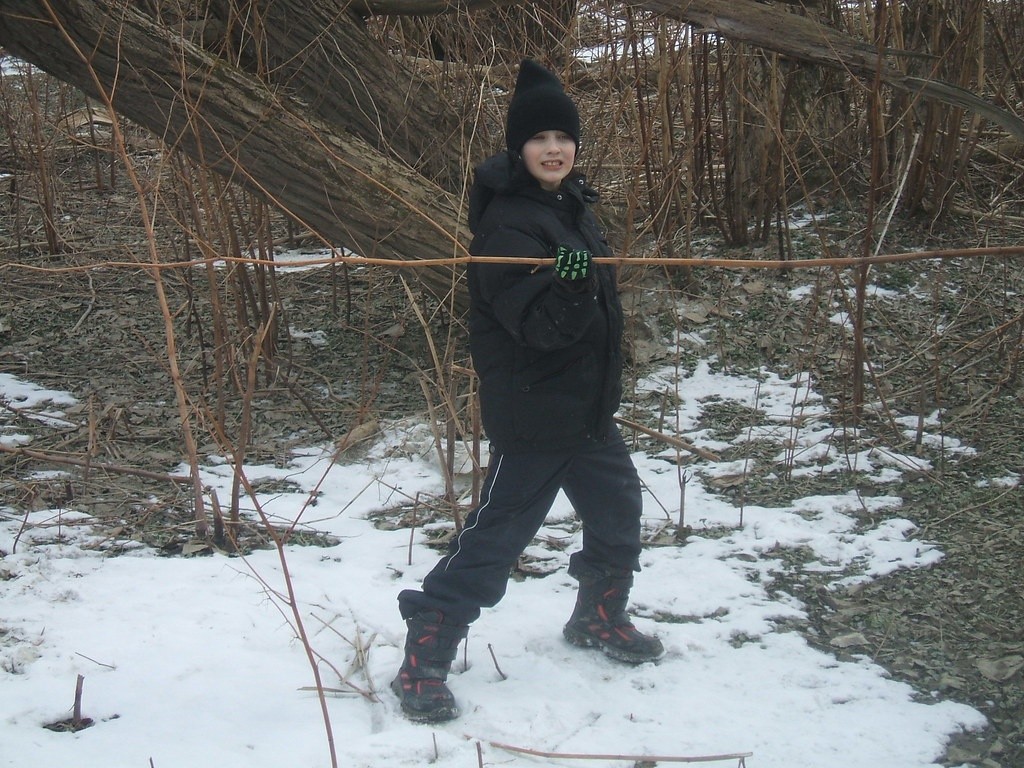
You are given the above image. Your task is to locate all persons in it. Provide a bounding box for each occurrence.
[390,58,666,723]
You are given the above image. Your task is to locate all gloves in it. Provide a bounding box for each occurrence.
[551,243,593,293]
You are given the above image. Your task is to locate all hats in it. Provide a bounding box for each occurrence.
[505,60,580,157]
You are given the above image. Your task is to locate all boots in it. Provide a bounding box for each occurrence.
[563,570,666,663]
[391,609,470,723]
